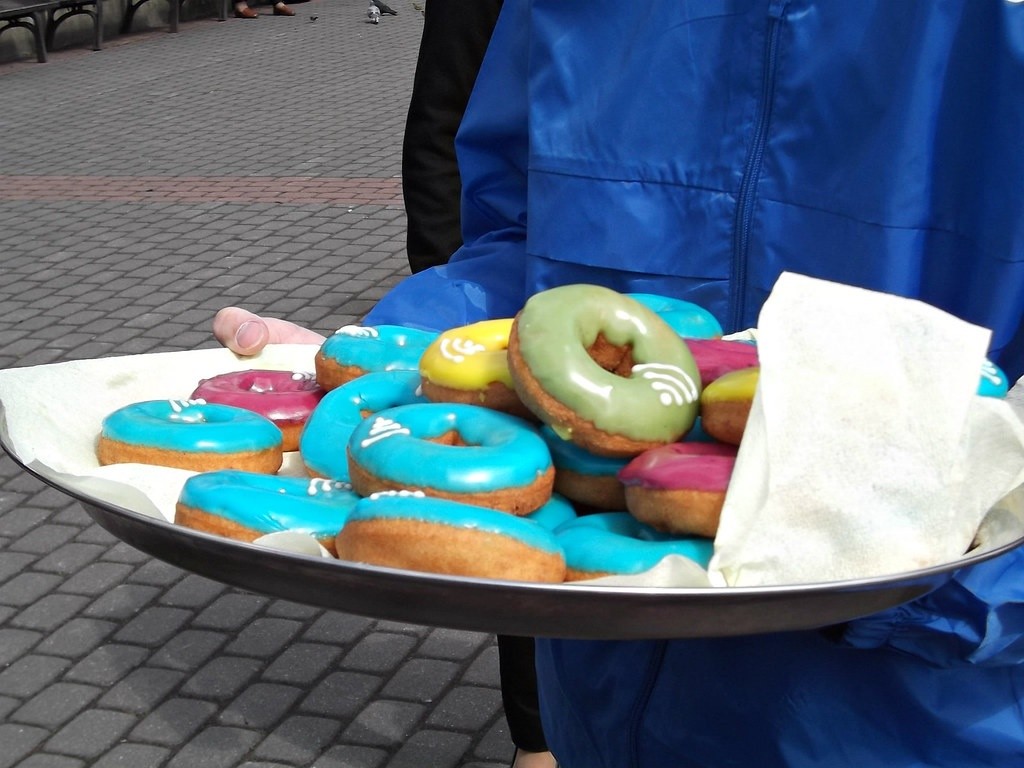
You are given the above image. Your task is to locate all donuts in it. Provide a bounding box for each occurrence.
[974,358,1010,398]
[90,284,759,586]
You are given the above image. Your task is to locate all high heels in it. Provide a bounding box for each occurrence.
[273,5,295,16]
[233,7,258,18]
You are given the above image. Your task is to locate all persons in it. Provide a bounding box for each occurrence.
[232,0,296,19]
[210,0,1024,768]
[401,1,559,768]
